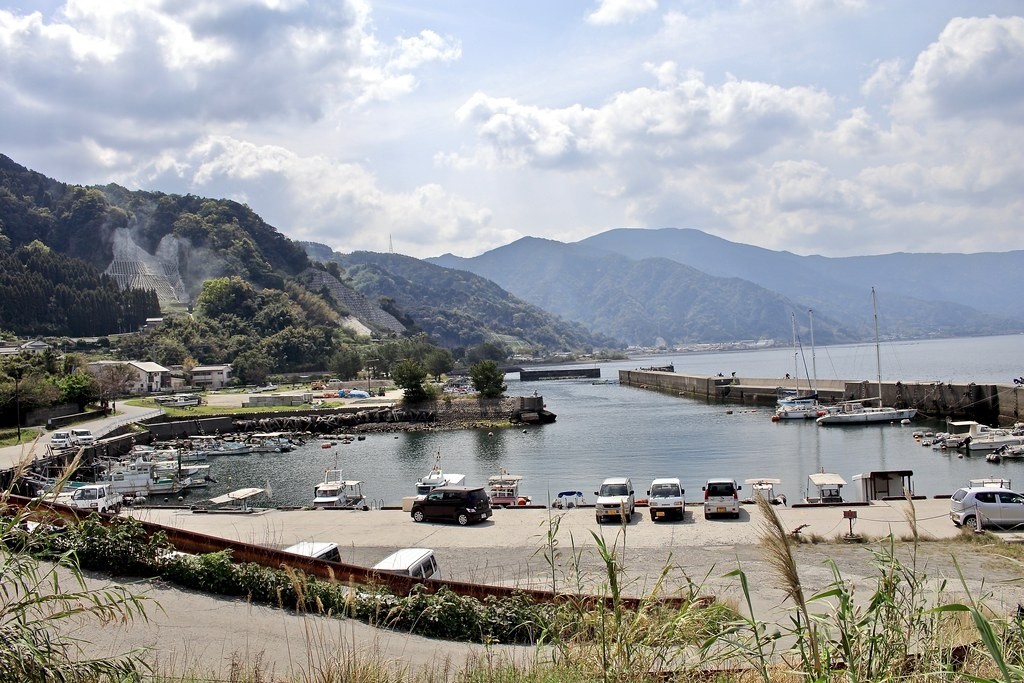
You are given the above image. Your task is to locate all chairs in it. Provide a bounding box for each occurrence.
[712,486,718,491]
[724,486,730,491]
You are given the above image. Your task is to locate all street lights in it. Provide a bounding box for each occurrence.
[365,359,380,395]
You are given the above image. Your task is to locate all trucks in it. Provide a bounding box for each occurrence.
[70,484,124,514]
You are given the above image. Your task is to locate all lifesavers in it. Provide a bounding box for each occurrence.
[962,392,967,397]
[897,396,901,399]
[778,388,782,393]
[933,405,937,409]
[896,390,901,395]
[933,400,937,405]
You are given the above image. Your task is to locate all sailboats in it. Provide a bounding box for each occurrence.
[774,285,918,426]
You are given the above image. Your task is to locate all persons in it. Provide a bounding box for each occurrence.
[1018,377,1024,387]
[719,373,722,377]
[732,372,736,377]
[786,372,791,379]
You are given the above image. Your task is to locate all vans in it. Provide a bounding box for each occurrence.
[593,477,635,524]
[646,477,686,523]
[371,549,442,581]
[702,479,742,521]
[411,486,493,526]
[281,541,343,563]
[948,486,1024,531]
[70,429,94,446]
[50,432,77,449]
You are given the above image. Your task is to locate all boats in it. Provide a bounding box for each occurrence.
[484,466,533,507]
[312,452,367,508]
[803,467,848,504]
[742,478,783,505]
[936,420,1024,458]
[414,446,466,499]
[27,432,292,496]
